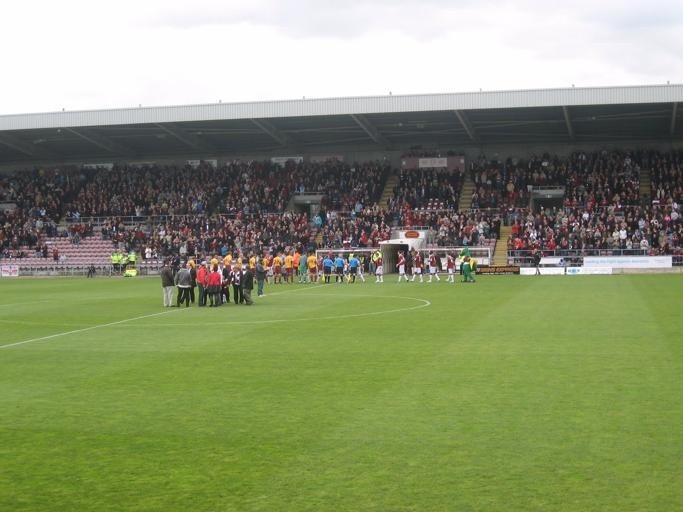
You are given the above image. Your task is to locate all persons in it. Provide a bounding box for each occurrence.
[2,146,683,308]
